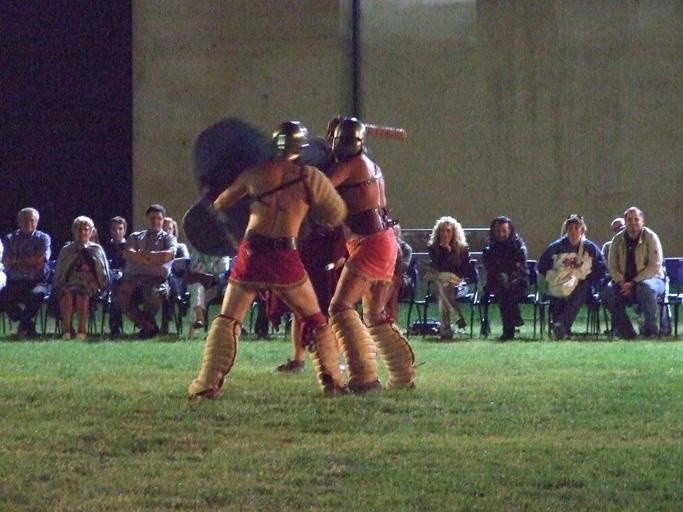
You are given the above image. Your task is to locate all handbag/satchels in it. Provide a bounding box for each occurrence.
[412,318,441,335]
[68,271,87,286]
[542,266,577,297]
[660,299,671,337]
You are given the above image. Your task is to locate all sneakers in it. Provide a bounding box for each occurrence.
[496,334,514,340]
[510,317,523,326]
[275,359,304,373]
[550,322,563,340]
[604,328,634,338]
[641,330,660,337]
[75,332,86,340]
[560,330,571,340]
[62,333,70,340]
[193,321,202,328]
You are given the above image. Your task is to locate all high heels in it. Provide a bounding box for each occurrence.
[455,319,466,331]
[439,336,452,341]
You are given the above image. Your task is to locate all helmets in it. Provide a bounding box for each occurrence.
[332,116,367,163]
[270,120,308,161]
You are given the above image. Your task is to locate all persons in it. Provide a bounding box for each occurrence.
[276,135,350,371]
[256,301,291,338]
[602,206,669,339]
[328,117,417,393]
[387,218,415,321]
[427,216,470,340]
[601,217,625,266]
[187,121,354,397]
[482,215,531,341]
[537,214,607,339]
[0,205,191,338]
[185,244,230,338]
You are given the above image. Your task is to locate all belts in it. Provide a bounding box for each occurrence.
[243,229,296,251]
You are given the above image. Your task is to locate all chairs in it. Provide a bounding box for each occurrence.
[393,253,683,342]
[1,258,290,340]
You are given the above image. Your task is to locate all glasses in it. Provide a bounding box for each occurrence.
[565,215,583,220]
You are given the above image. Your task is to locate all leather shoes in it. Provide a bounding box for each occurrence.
[17,323,27,337]
[26,321,34,336]
[138,329,144,336]
[144,326,158,336]
[110,328,122,336]
